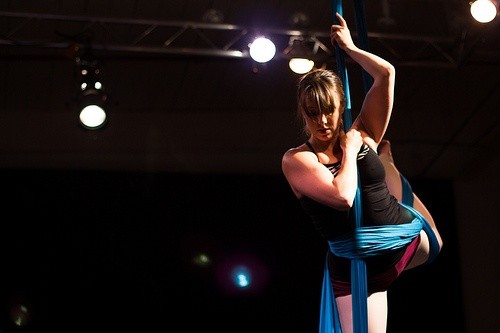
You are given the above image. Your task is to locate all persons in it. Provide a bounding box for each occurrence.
[282,12,442,333]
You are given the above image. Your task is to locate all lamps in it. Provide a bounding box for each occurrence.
[469,0,499,24]
[79,87,107,129]
